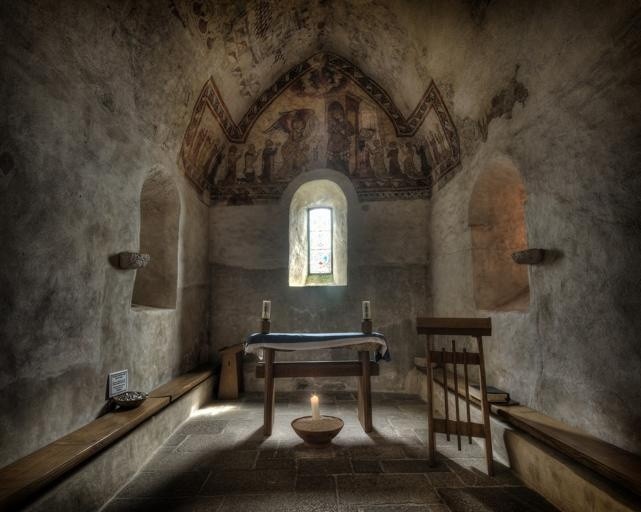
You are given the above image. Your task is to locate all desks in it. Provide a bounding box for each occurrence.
[248,330,388,434]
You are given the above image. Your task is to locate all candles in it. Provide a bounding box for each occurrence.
[311,394,321,422]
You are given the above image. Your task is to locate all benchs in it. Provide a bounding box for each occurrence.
[416,362,640,510]
[0,363,223,512]
